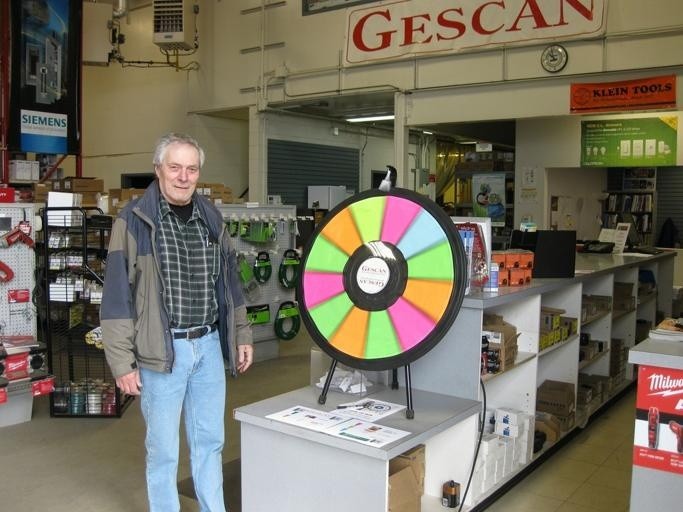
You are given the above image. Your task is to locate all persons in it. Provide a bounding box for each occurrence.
[99,131,254,512]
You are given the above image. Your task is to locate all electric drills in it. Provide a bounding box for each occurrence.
[0,227,35,248]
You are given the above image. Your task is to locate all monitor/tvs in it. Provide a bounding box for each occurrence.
[620,212,641,252]
[507,230,577,279]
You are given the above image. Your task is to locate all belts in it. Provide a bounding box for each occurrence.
[174,324,217,341]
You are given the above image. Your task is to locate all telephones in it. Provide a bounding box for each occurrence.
[577,240,615,254]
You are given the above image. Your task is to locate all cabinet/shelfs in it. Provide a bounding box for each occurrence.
[232,379,481,512]
[0,201,297,427]
[625,319,683,512]
[393,248,683,512]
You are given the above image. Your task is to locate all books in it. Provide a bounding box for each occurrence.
[649,316,683,342]
[603,194,652,247]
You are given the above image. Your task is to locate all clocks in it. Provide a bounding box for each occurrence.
[541,45,568,73]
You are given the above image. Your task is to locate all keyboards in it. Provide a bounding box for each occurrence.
[638,249,664,254]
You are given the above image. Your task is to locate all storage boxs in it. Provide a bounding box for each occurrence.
[8,160,233,215]
